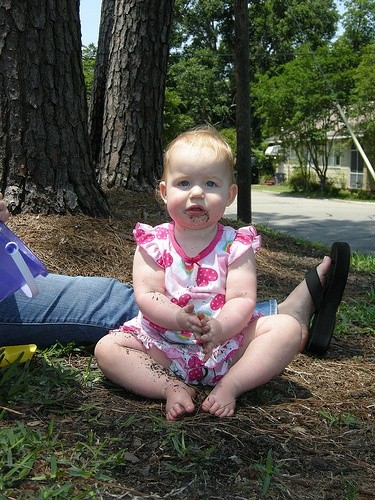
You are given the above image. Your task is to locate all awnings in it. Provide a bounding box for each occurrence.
[264,145,282,157]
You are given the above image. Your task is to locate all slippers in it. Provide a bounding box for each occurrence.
[305,241,351,361]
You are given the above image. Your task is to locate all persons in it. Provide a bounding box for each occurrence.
[0,192,351,358]
[93,124,302,420]
